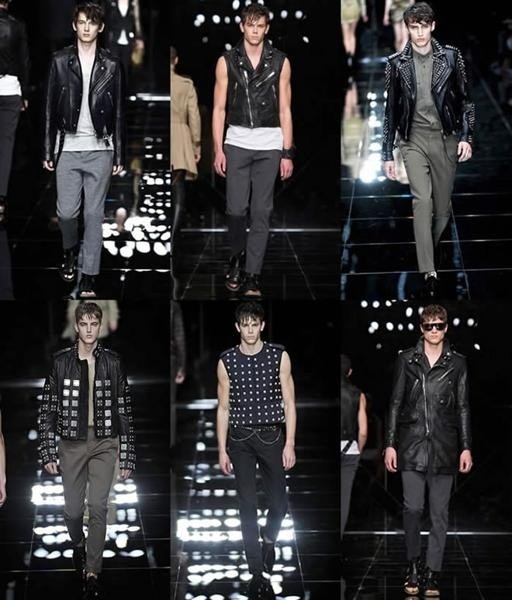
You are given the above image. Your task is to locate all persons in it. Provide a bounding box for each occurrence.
[340,0,369,67]
[38,303,136,597]
[382,0,415,52]
[42,2,124,298]
[381,2,477,295]
[213,4,294,296]
[216,300,298,589]
[0,0,30,221]
[171,301,187,448]
[340,354,368,540]
[383,304,475,597]
[170,46,202,258]
[0,409,7,508]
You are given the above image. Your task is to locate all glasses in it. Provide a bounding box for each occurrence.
[421,321,447,331]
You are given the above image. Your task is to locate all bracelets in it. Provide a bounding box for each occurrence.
[282,149,294,159]
